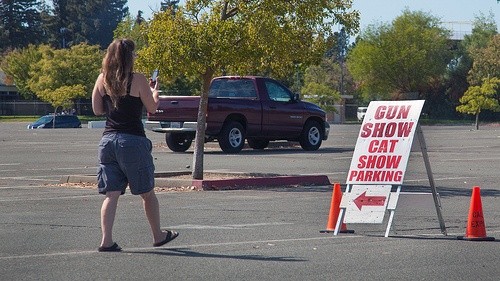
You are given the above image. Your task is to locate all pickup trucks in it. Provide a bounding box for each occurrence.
[144,75,331,153]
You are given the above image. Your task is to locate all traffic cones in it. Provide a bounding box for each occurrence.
[318,182,356,233]
[457,185,497,242]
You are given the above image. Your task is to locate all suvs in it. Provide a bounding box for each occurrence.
[27,114,83,129]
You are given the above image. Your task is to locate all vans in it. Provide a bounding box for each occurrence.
[356,107,367,122]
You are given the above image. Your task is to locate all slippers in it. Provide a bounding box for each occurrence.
[97,242,124,252]
[153,230,179,246]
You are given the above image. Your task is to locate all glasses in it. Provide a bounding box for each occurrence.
[132,53,139,58]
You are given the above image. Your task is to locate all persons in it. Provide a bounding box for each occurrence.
[91,39,180,252]
